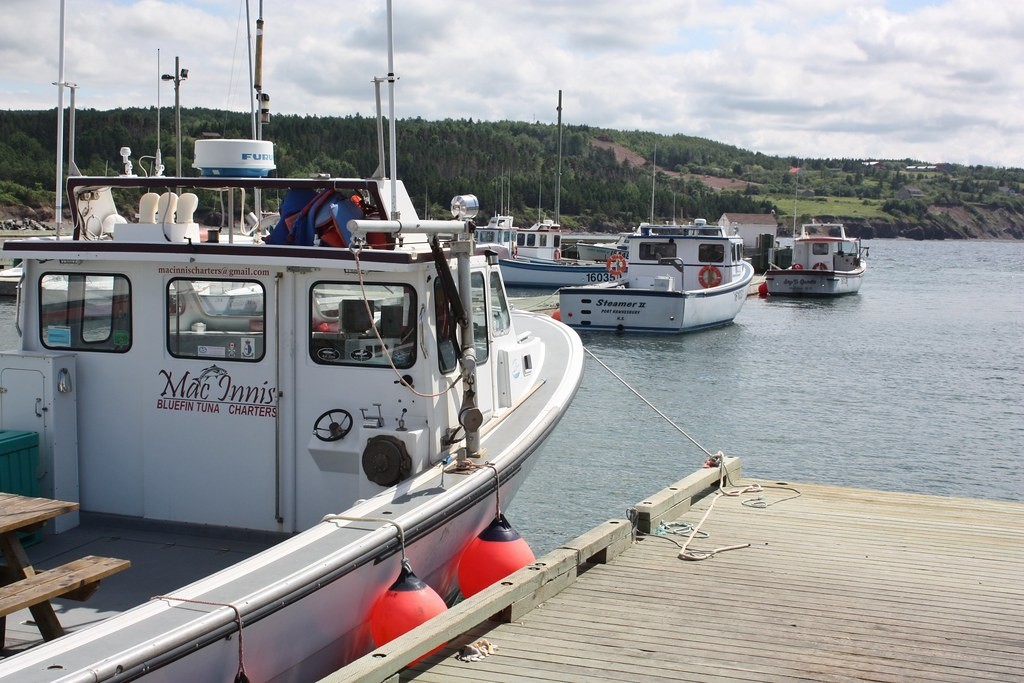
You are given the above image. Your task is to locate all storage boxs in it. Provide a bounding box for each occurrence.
[0,427,46,551]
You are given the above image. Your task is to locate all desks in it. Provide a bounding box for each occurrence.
[0,490,81,655]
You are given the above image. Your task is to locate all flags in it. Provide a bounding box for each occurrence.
[788,168,800,173]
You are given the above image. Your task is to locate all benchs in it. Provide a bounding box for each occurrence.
[1,554,133,619]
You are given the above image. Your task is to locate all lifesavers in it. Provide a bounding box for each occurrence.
[812,263,827,270]
[606,254,626,275]
[698,264,721,287]
[554,249,561,259]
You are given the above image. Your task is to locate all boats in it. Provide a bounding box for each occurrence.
[767,223,869,298]
[559,217,756,332]
[0,134,588,683]
[0,0,661,332]
